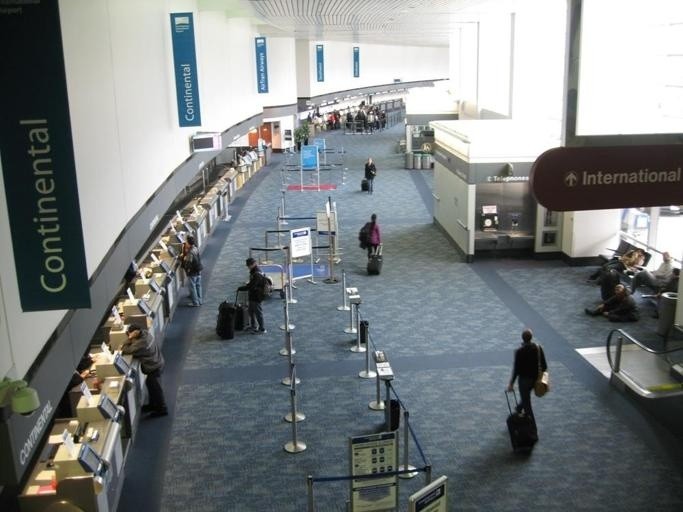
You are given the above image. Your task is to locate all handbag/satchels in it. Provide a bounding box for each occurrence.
[534,372,549,397]
[184,255,203,275]
[360,241,369,249]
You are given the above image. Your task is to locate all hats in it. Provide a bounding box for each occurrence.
[246,258,256,266]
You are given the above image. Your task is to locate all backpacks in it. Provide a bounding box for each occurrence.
[255,272,273,296]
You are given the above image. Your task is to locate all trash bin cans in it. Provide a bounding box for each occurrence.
[405,152,431,170]
[654,292,678,337]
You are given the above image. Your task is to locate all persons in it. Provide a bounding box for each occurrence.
[238,257,267,334]
[67,370,89,390]
[359,214,383,258]
[584,248,679,322]
[182,235,202,307]
[122,325,168,418]
[507,329,547,440]
[365,157,376,195]
[308,106,386,132]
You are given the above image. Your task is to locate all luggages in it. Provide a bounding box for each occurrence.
[362,180,369,191]
[367,244,384,275]
[601,269,620,299]
[216,289,249,340]
[505,389,538,449]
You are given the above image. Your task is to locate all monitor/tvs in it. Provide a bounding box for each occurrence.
[97,392,121,422]
[78,443,101,476]
[138,222,194,316]
[113,353,129,375]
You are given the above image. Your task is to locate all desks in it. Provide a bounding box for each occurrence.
[17,150,262,512]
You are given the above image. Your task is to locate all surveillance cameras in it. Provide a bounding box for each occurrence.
[10,380,40,417]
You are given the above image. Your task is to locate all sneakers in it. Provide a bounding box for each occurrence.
[249,328,267,335]
[188,303,200,306]
[141,404,168,418]
